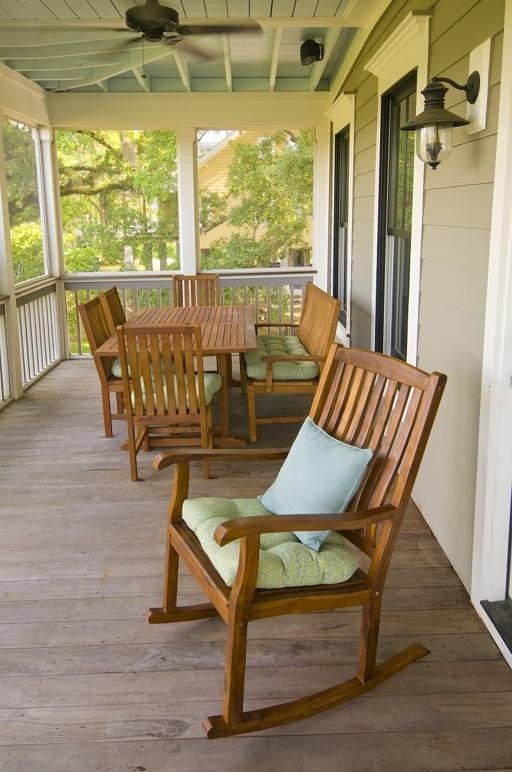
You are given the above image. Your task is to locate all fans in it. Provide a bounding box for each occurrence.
[40,0,264,63]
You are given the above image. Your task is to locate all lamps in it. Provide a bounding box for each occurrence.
[400,71,480,170]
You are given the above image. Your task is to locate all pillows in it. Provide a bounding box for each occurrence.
[258,416,374,553]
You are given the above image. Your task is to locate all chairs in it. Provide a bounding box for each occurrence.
[145,342,448,742]
